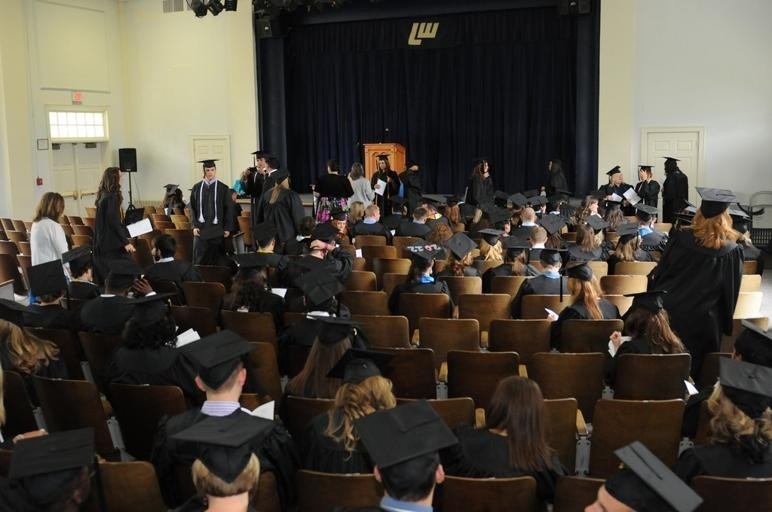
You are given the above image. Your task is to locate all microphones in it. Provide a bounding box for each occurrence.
[379,128,389,144]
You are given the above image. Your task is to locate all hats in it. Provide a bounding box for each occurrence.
[197,160,221,178]
[232,210,349,304]
[544,146,570,168]
[252,150,282,162]
[311,318,361,342]
[607,166,620,176]
[471,156,496,167]
[695,187,738,216]
[8,426,95,502]
[26,258,66,296]
[325,347,395,384]
[736,318,771,361]
[176,328,256,382]
[123,293,177,328]
[375,154,391,160]
[638,165,654,171]
[624,290,668,311]
[716,354,772,418]
[605,439,705,512]
[389,191,657,280]
[172,414,275,483]
[104,258,142,286]
[662,157,680,168]
[352,398,462,487]
[62,247,91,270]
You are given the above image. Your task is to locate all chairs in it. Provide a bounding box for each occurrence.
[0,206,772,512]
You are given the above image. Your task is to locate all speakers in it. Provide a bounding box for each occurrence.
[118,148,138,173]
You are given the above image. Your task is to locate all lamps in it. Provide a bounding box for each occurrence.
[185,0,237,17]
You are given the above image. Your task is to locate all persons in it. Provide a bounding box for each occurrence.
[312,159,354,224]
[369,153,400,214]
[540,155,569,215]
[398,159,423,215]
[345,162,377,210]
[240,149,271,196]
[468,159,495,204]
[189,158,236,267]
[1,185,771,512]
[623,164,661,220]
[95,165,138,288]
[260,156,280,197]
[659,157,689,220]
[597,166,633,217]
[255,169,305,252]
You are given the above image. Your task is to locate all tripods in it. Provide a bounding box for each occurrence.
[126,174,137,211]
